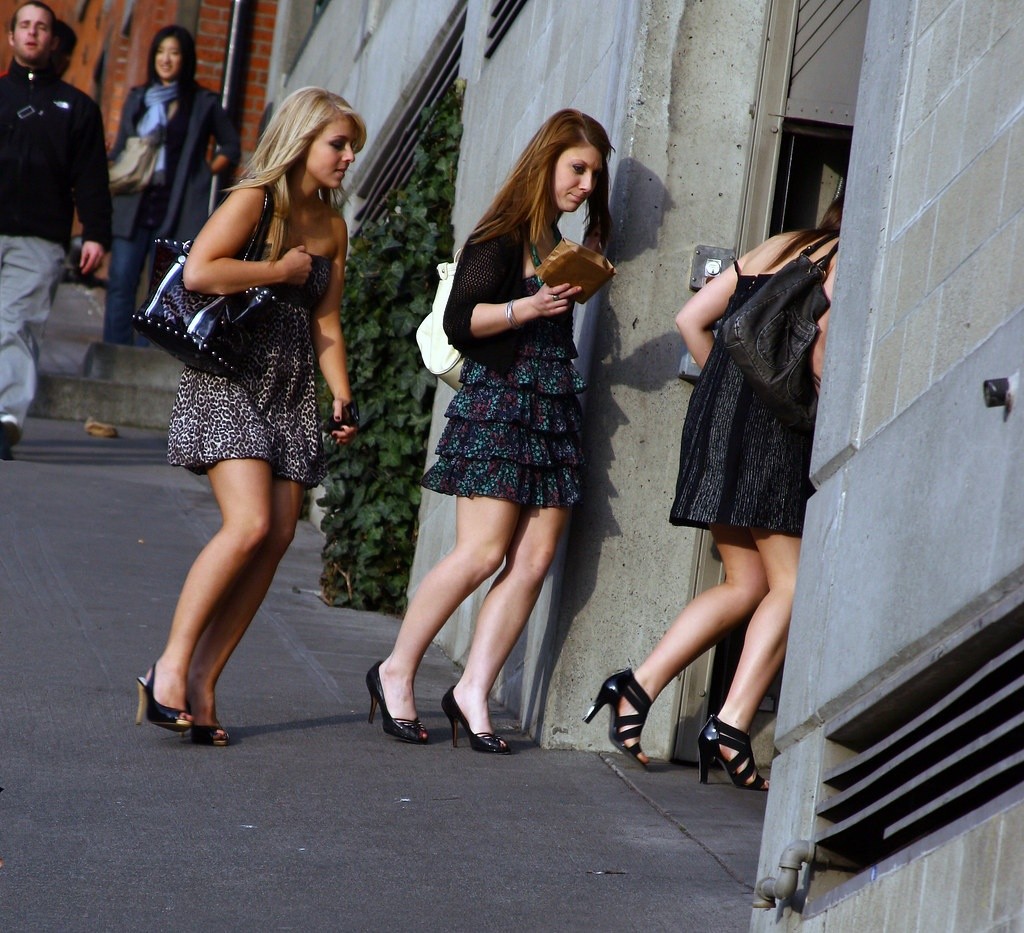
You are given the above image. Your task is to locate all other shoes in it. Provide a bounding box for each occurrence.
[0,421,22,461]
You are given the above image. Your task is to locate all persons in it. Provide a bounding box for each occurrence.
[0,1,114,460]
[104,24,240,349]
[134,86,363,747]
[583,192,846,794]
[366,108,615,755]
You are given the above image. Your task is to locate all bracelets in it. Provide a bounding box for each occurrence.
[505,300,523,328]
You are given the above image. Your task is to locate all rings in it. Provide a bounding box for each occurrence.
[553,295,559,301]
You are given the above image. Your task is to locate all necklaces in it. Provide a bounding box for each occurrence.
[527,241,546,287]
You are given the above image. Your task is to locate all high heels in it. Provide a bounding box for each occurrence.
[581,667,654,773]
[186,700,231,747]
[135,662,194,733]
[441,685,512,755]
[696,714,770,792]
[366,660,430,744]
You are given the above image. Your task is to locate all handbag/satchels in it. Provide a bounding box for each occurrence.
[722,232,842,437]
[109,136,159,194]
[415,246,466,393]
[129,179,276,377]
